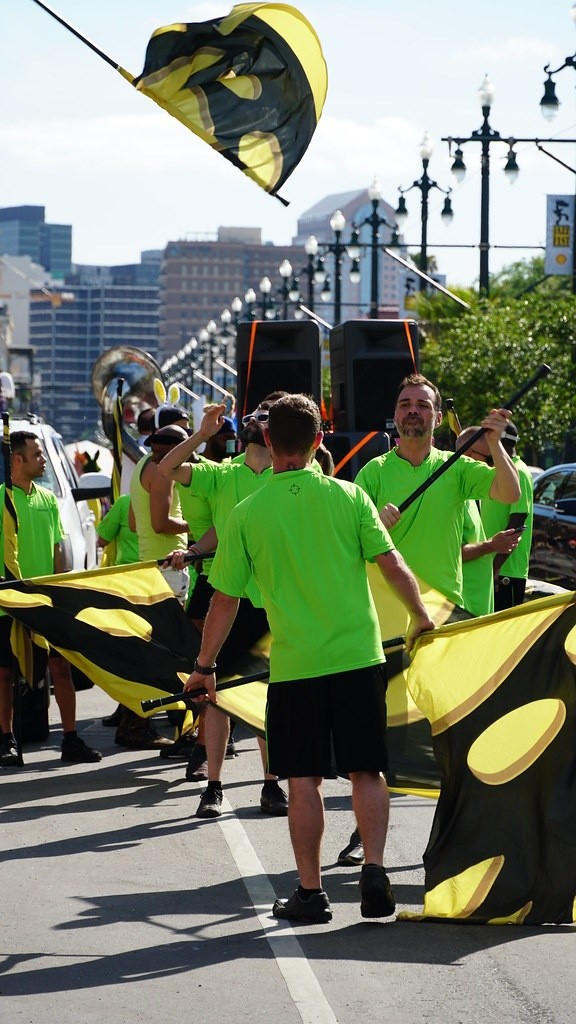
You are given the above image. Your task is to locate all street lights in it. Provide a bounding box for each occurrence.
[438,71,521,315]
[160,208,360,412]
[538,0,576,299]
[343,175,403,320]
[392,132,454,294]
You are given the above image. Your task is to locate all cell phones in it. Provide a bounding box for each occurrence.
[513,525,528,533]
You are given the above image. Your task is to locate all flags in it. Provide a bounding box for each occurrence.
[0,567,188,719]
[394,593,576,925]
[133,0,328,195]
[184,564,477,798]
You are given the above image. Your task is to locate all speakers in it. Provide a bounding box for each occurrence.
[233,316,419,480]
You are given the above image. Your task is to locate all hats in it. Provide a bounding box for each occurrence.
[158,406,189,427]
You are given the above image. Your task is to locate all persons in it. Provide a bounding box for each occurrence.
[157,390,324,818]
[479,420,533,611]
[182,394,436,924]
[0,396,236,769]
[443,426,523,616]
[337,374,523,866]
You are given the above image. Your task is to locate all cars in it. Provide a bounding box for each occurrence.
[528,463,575,593]
[0,415,99,691]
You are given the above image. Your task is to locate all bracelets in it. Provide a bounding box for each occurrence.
[189,544,206,555]
[194,659,217,675]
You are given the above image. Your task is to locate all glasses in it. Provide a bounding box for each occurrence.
[472,447,495,466]
[242,412,270,424]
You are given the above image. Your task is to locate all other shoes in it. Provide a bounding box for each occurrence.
[224,738,236,759]
[115,720,174,749]
[101,703,152,726]
[160,732,197,758]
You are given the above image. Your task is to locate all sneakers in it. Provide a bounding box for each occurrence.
[338,829,365,863]
[0,737,24,770]
[259,783,288,813]
[196,785,223,817]
[273,889,332,923]
[186,751,208,778]
[359,864,395,917]
[63,738,102,763]
